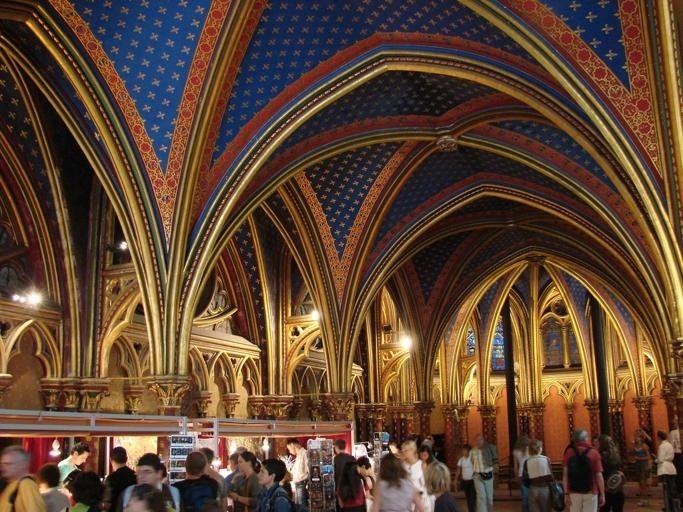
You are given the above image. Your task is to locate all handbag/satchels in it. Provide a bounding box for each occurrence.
[549,476,567,512]
[479,471,493,480]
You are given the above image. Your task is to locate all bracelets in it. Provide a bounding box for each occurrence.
[563,493,569,495]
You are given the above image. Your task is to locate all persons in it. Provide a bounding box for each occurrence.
[521,439,556,512]
[627,430,651,507]
[512,437,530,512]
[591,436,600,447]
[453,445,477,511]
[1,437,311,512]
[654,431,678,512]
[561,428,606,512]
[669,425,682,498]
[597,434,625,512]
[468,433,494,512]
[333,434,452,512]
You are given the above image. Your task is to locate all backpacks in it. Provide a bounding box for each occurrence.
[269,491,310,512]
[566,446,594,494]
